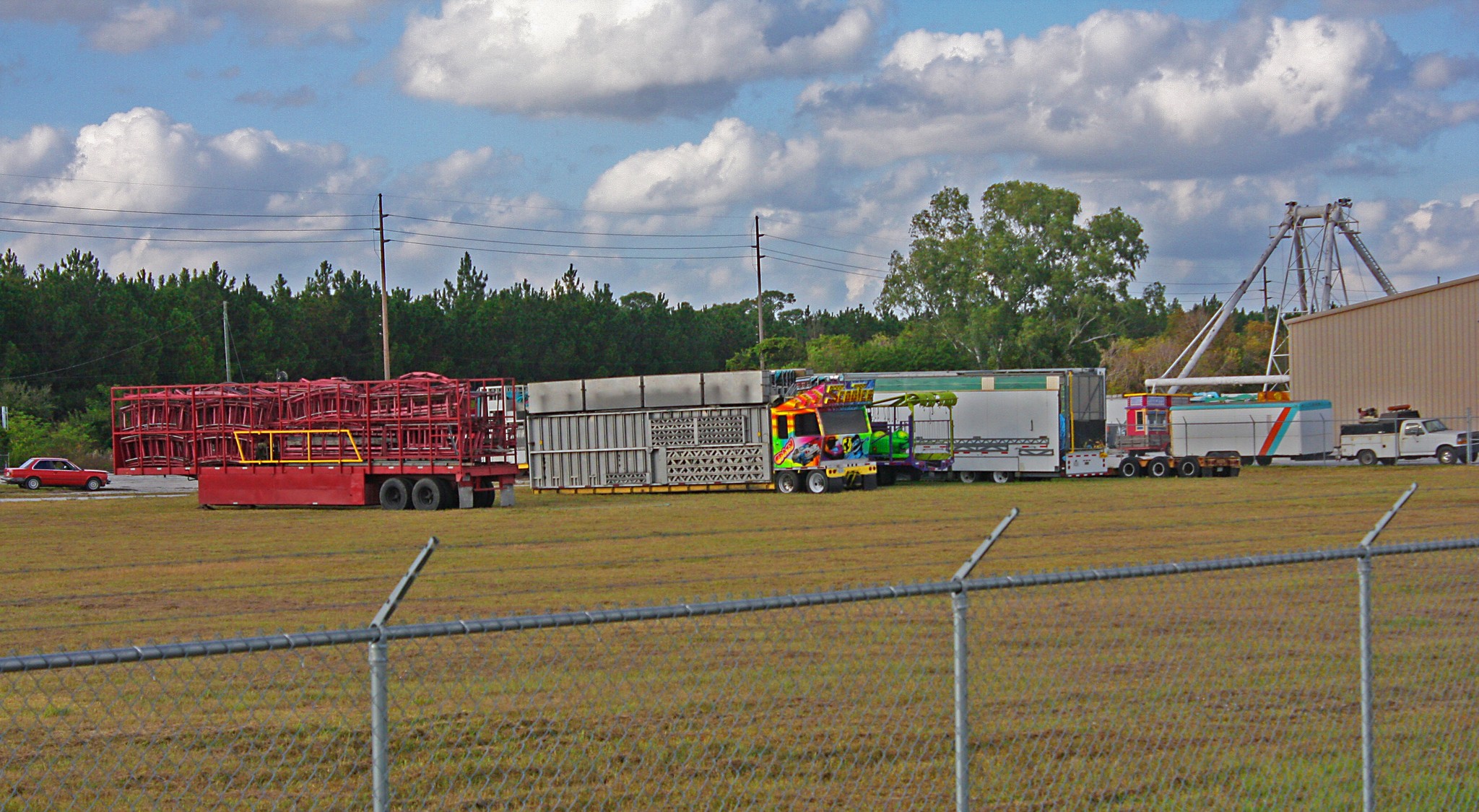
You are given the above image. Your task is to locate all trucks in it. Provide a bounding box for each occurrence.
[771,382,877,495]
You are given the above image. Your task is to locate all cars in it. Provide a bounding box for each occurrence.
[0,457,111,491]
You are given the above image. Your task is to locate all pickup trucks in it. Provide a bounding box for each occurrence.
[1334,417,1479,467]
